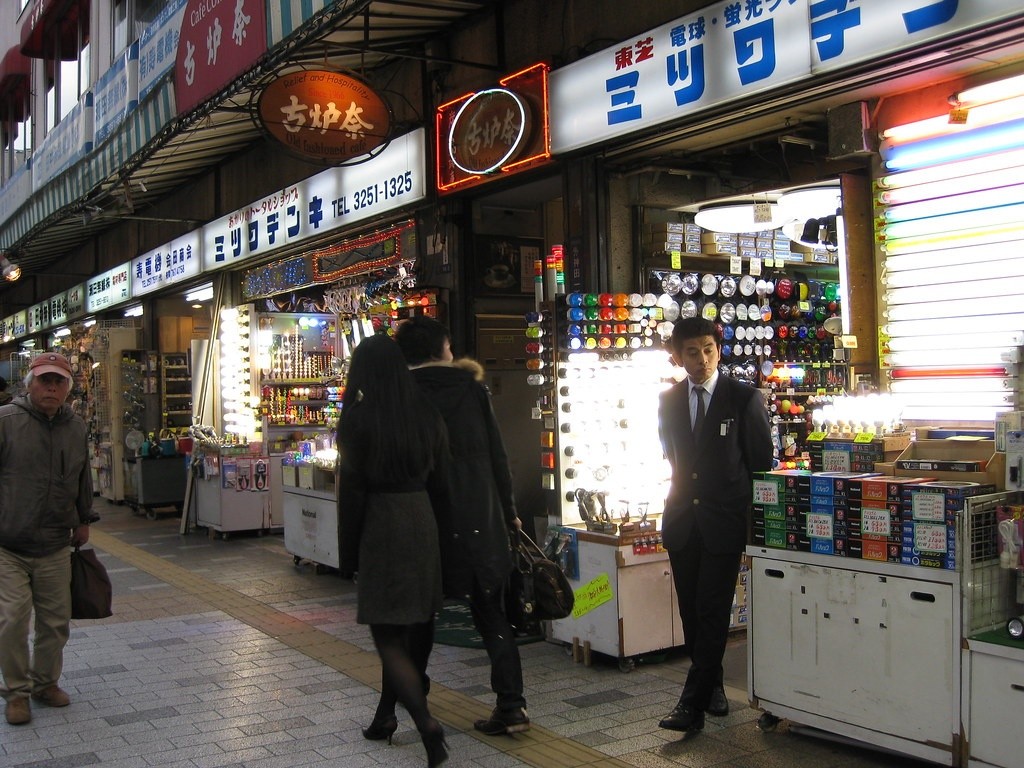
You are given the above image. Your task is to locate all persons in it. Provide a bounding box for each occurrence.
[0,352,100,723]
[336,318,531,768]
[0,376,13,407]
[659,317,774,731]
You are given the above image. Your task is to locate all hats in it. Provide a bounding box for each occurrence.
[30,352,72,381]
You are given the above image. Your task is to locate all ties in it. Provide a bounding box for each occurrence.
[691,388,706,445]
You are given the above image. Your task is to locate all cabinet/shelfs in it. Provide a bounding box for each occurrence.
[159,352,193,428]
[549,522,685,673]
[194,457,270,541]
[259,380,328,430]
[744,545,962,768]
[281,485,339,573]
[961,625,1024,768]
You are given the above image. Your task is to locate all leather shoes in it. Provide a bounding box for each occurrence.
[658,695,704,731]
[473,706,532,735]
[704,687,729,716]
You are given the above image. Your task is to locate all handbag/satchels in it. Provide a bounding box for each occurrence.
[504,529,574,624]
[70,548,113,619]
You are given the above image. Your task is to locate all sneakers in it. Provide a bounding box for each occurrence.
[31,685,71,705]
[5,695,31,724]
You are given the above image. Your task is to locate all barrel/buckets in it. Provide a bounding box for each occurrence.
[178,437,193,455]
[160,438,176,456]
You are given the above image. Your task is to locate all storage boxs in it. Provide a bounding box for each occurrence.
[157,304,210,353]
[279,465,298,487]
[747,426,1005,571]
[642,222,838,265]
[730,552,747,626]
[297,466,330,491]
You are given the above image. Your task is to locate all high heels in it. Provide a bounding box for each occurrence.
[417,717,449,768]
[362,716,397,745]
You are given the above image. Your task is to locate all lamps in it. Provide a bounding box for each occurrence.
[694,200,782,233]
[777,185,841,215]
[879,74,1024,422]
[783,215,837,250]
[835,206,850,334]
[0,248,22,282]
